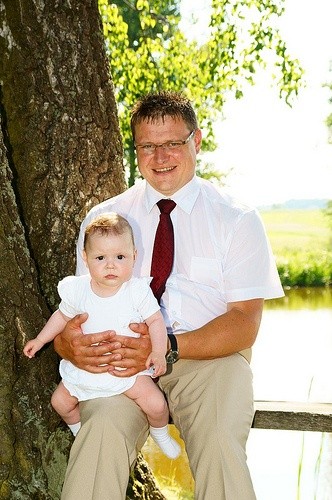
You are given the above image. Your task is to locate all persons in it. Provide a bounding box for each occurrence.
[54,89,285,500]
[23,212,181,460]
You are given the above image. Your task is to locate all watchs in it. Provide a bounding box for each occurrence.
[165,333,180,364]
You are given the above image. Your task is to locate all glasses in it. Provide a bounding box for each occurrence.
[133,129,196,155]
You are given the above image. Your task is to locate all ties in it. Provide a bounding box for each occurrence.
[149,199,176,305]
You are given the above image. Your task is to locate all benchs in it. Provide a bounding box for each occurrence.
[250,400,332,433]
